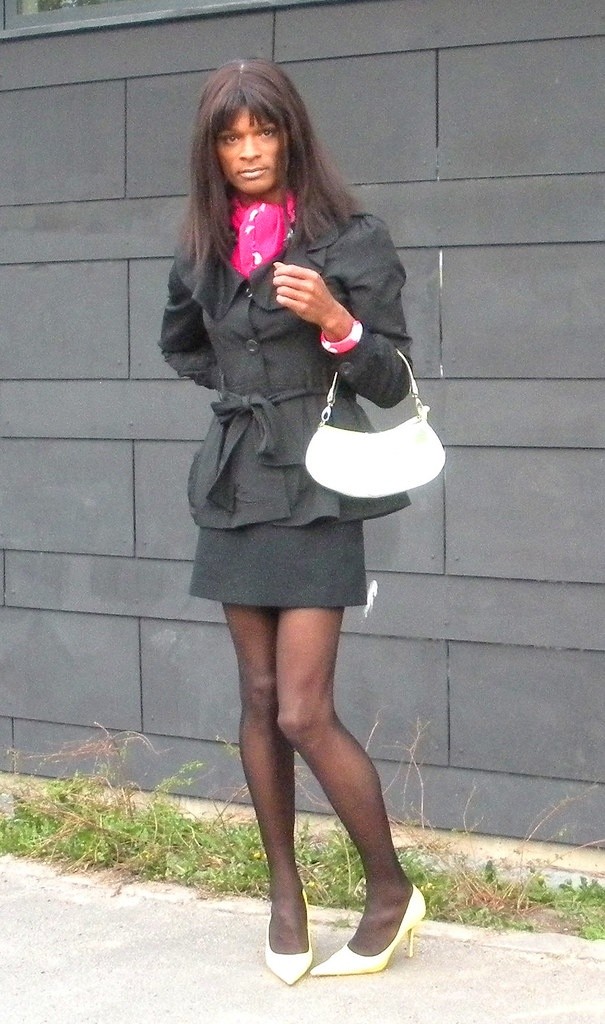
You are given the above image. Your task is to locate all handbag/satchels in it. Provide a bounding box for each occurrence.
[305,350,446,498]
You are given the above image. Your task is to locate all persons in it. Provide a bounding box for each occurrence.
[157,58,428,983]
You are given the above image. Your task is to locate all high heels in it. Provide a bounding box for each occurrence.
[266,890,314,987]
[310,884,427,977]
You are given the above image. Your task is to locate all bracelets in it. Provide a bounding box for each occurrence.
[320,321,363,354]
